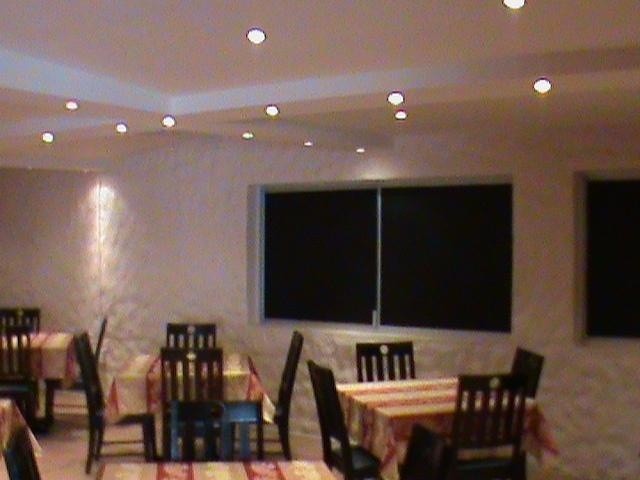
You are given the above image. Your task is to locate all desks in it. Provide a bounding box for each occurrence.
[94,458,349,480]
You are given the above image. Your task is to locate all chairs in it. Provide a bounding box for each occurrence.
[305,340,545,480]
[0,301,306,475]
